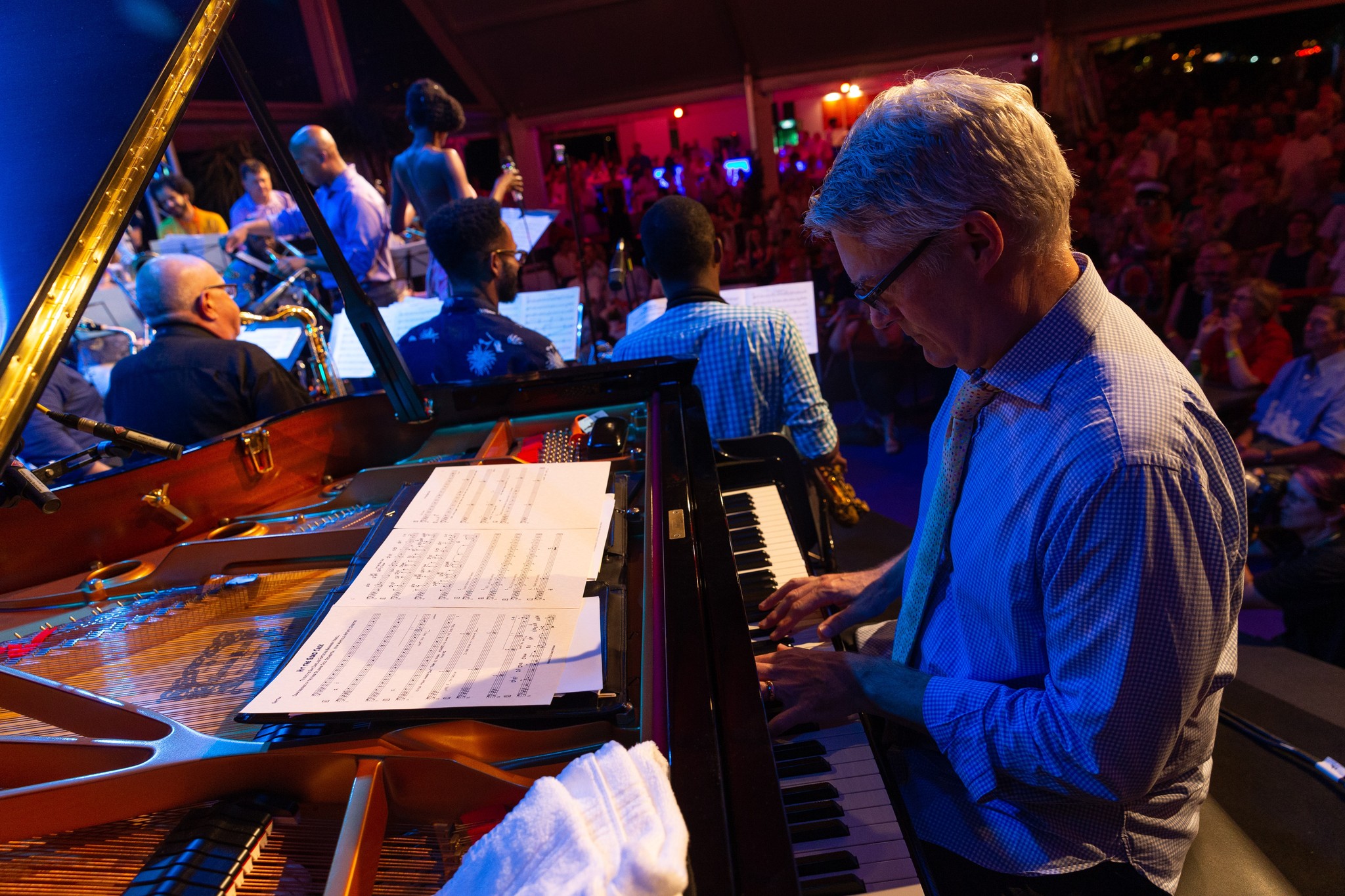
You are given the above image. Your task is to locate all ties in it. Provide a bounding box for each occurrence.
[892,385,997,671]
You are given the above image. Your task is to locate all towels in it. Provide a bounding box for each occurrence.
[438,736,696,895]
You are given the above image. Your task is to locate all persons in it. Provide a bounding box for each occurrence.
[754,67,1248,896]
[390,79,525,300]
[105,252,313,467]
[224,125,398,314]
[395,196,568,386]
[611,197,849,475]
[524,70,1345,663]
[91,174,229,323]
[16,361,122,478]
[228,158,313,244]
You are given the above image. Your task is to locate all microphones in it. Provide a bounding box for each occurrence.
[500,156,523,203]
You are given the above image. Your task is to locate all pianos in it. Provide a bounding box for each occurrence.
[0,0,931,894]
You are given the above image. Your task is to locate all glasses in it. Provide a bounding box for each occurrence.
[205,284,238,300]
[490,248,528,267]
[854,236,935,312]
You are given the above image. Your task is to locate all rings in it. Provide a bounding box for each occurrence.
[765,680,775,701]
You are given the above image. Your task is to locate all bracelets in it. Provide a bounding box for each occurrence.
[827,454,836,462]
